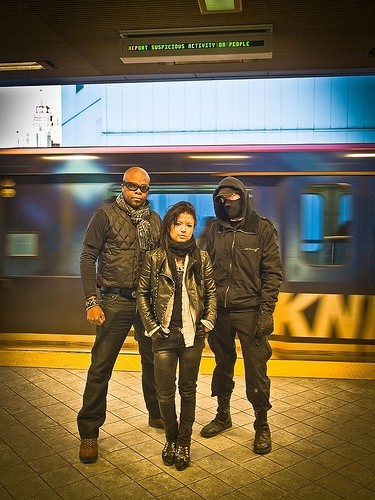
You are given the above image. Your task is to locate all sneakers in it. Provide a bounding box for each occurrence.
[176,444,190,469]
[161,442,174,466]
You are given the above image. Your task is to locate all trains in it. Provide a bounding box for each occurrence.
[0,142,375,362]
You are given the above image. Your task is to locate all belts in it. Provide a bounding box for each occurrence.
[100,284,136,298]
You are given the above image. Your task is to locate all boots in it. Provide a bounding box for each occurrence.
[252,410,272,454]
[199,391,240,443]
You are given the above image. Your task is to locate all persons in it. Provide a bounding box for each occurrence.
[77,167,163,463]
[136,201,217,471]
[200,177,283,454]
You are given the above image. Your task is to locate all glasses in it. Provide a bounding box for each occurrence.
[123,180,150,192]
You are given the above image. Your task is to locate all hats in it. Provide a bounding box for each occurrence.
[213,186,238,200]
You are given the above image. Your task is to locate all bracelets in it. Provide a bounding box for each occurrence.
[86,298,99,311]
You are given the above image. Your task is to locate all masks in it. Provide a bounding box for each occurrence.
[222,198,243,218]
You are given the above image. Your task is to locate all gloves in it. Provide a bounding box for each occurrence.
[252,312,274,339]
[195,321,208,339]
[152,329,169,342]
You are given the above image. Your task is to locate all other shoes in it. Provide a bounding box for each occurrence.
[80,438,98,462]
[149,416,165,429]
